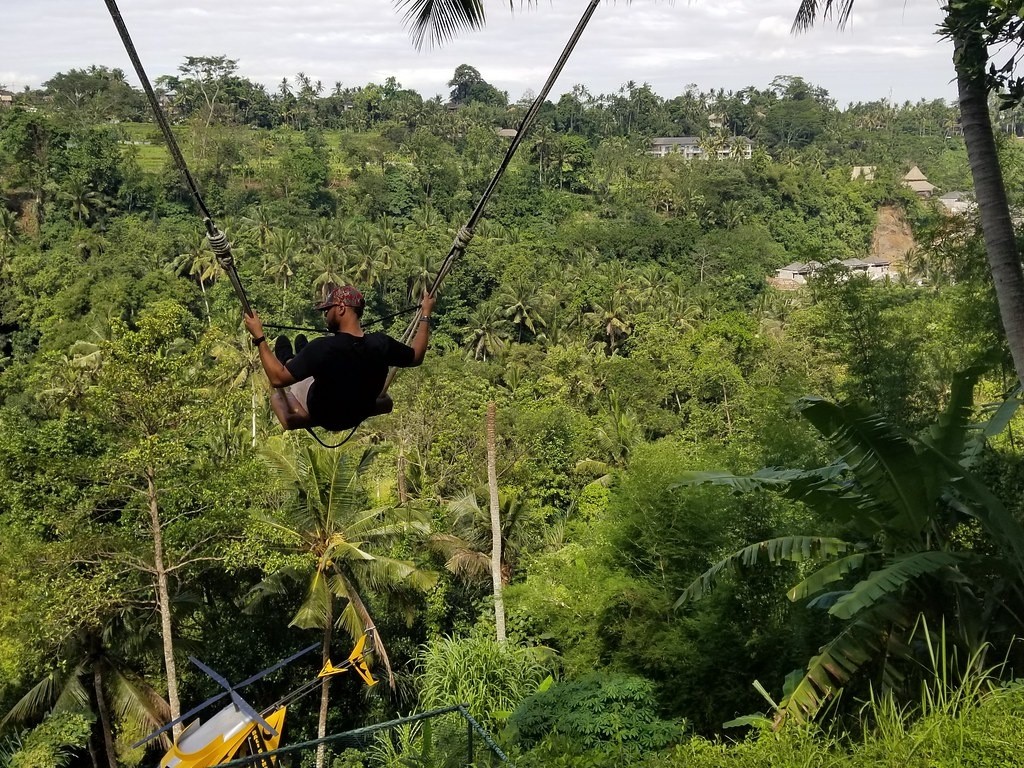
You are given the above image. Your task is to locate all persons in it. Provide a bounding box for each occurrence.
[245,285,436,430]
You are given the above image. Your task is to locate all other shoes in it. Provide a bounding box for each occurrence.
[274,335,296,366]
[295,333,308,354]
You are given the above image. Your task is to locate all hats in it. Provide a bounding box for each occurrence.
[318,286,366,309]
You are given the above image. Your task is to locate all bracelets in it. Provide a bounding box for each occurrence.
[419,315,430,321]
[252,337,265,346]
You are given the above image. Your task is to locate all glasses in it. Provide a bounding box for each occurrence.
[324,304,336,317]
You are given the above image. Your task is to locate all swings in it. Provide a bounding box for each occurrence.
[105,0,604,433]
